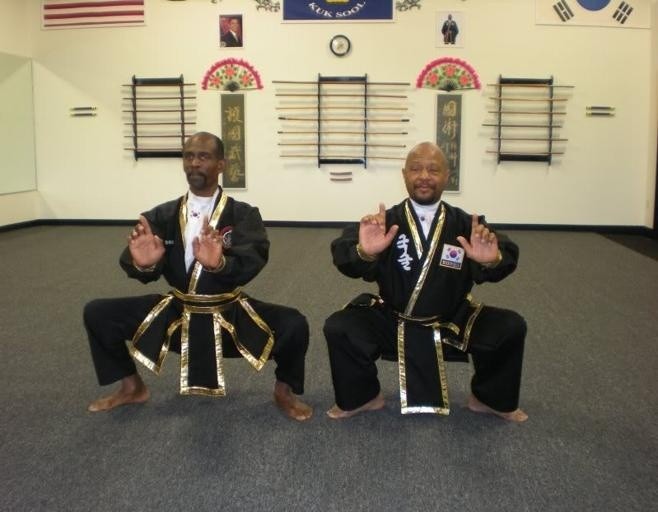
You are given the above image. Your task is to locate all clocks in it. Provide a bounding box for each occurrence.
[329,34,352,58]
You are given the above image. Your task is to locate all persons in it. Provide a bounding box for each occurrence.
[322,142,528,421]
[81,131,312,422]
[440,13,459,42]
[221,17,239,46]
